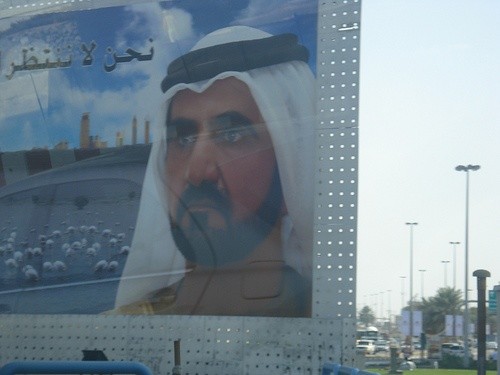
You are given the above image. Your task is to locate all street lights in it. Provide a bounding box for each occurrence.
[374,277,407,316]
[418,269,427,299]
[441,260,450,287]
[455,163,481,344]
[404,220,419,334]
[450,241,461,314]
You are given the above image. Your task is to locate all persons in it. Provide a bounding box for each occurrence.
[96,24,316,318]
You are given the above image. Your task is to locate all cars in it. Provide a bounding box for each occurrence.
[356,326,497,359]
